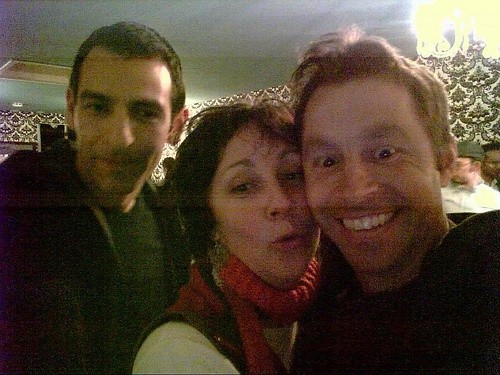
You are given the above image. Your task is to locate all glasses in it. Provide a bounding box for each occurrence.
[455,162,471,170]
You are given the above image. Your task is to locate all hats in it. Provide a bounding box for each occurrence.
[457,141,485,161]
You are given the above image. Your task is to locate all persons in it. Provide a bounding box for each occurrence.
[0,22,189,375]
[441,141,500,212]
[132,97,321,375]
[289,24,500,375]
